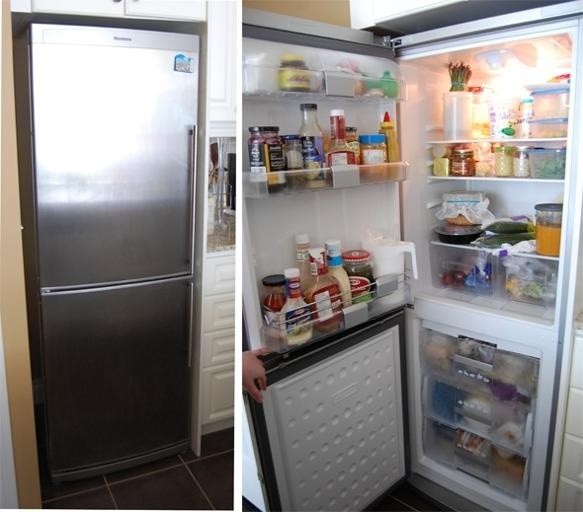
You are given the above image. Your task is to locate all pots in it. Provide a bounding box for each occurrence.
[436,232,481,245]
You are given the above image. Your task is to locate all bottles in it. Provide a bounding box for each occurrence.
[303,247,345,333]
[494,146,512,177]
[378,111,400,162]
[345,126,361,165]
[278,54,311,91]
[280,134,304,188]
[297,103,327,189]
[262,274,286,330]
[342,249,375,304]
[293,233,311,288]
[325,238,353,307]
[247,125,286,194]
[279,267,313,345]
[513,149,530,178]
[324,109,355,167]
[452,149,475,177]
[358,133,387,165]
[534,203,562,256]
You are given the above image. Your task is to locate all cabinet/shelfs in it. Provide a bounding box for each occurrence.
[12,0,207,21]
[191,5,239,458]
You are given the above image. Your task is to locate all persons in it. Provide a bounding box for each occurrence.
[242,348,274,403]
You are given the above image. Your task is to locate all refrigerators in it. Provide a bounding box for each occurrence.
[28,21,200,485]
[244,2,582,507]
